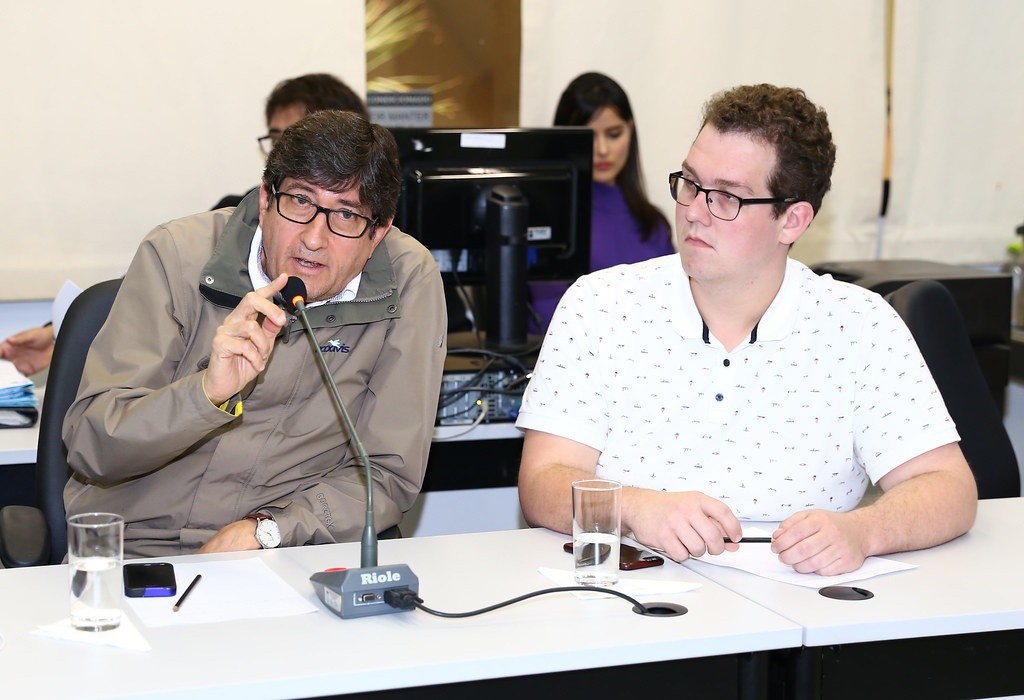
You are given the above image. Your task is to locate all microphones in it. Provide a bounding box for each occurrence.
[277,274,419,620]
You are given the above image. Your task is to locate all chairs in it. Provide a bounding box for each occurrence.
[37,279,126,556]
[880,283,1020,499]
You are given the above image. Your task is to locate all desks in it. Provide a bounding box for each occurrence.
[0,381,527,571]
[0,497,1024,700]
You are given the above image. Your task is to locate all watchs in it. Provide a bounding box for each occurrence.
[242,514,281,550]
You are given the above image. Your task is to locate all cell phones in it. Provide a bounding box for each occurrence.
[124,563,176,599]
[563,542,664,571]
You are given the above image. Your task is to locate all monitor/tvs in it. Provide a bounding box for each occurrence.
[381,126,593,373]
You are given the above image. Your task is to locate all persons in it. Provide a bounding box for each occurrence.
[513,84,978,576]
[0,71,369,378]
[59,110,447,563]
[521,72,675,334]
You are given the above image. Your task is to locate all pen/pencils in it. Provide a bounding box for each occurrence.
[173,575,202,612]
[723,537,776,544]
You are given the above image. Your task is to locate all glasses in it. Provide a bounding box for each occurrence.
[271,184,380,239]
[257,135,279,156]
[668,171,809,221]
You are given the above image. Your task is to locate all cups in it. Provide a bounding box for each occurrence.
[66,512,126,632]
[571,479,622,588]
